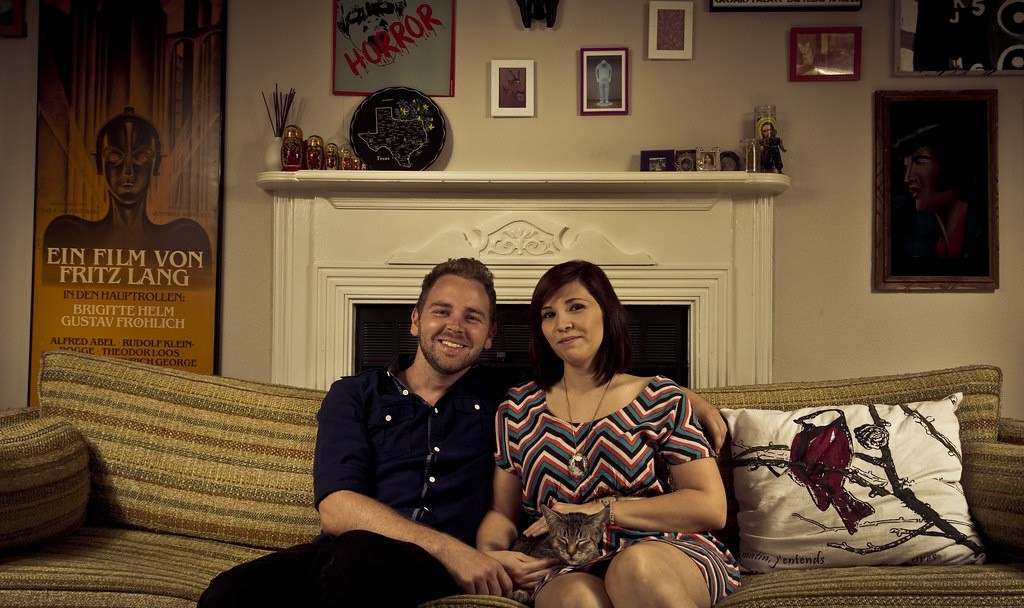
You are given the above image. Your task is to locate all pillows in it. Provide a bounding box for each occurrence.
[720,391,988,574]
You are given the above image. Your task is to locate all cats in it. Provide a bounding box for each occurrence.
[509,503,610,605]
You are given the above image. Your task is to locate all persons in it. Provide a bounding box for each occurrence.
[477,262,731,607]
[201,257,511,607]
[702,153,715,168]
[762,129,788,176]
[651,159,666,169]
[681,159,690,170]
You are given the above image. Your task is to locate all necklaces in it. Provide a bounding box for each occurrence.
[563,374,612,481]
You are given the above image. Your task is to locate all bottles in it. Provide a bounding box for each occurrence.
[738,104,777,173]
[265,137,283,170]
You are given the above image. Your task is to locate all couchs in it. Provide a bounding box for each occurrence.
[0,351,1024,608]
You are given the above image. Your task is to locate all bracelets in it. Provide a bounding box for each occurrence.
[605,498,618,523]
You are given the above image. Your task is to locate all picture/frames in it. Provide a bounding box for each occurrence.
[894,0,1024,77]
[790,27,861,81]
[696,146,721,171]
[490,59,534,118]
[579,47,629,116]
[641,148,696,171]
[332,0,457,97]
[27,0,226,408]
[871,89,1000,290]
[648,1,694,59]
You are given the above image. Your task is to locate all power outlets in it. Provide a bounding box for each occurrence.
[0,0,28,38]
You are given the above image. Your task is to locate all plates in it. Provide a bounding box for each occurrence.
[349,86,446,170]
